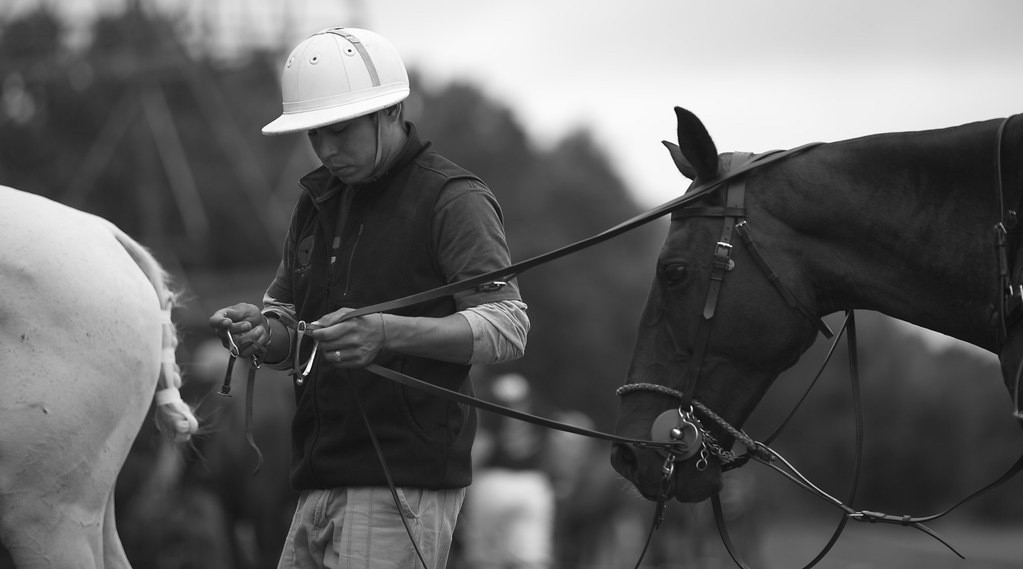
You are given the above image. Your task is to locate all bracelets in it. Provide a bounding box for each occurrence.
[260,315,272,349]
[379,312,385,351]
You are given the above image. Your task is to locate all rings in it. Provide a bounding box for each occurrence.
[333,351,340,363]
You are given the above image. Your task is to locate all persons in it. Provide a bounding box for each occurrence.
[207,26,529,569]
[447,375,757,569]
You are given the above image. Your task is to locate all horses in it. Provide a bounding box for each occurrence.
[0,183,202,568]
[608,106,1023,504]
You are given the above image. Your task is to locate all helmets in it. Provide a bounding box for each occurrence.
[261,27,411,137]
